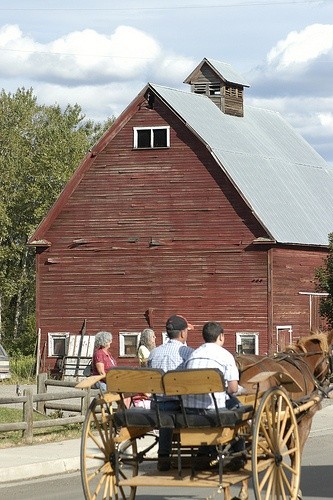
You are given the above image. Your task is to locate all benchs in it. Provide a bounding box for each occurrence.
[107,367,253,484]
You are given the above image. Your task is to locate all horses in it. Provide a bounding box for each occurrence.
[234,328,333,500]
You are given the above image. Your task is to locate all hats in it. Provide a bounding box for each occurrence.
[166,315,194,331]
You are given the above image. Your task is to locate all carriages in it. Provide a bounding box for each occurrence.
[79,328,333,500]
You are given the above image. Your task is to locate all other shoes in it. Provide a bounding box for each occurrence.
[158,458,170,471]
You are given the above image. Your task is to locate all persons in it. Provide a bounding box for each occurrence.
[136,329,157,367]
[92,331,118,408]
[148,315,195,400]
[181,322,247,471]
